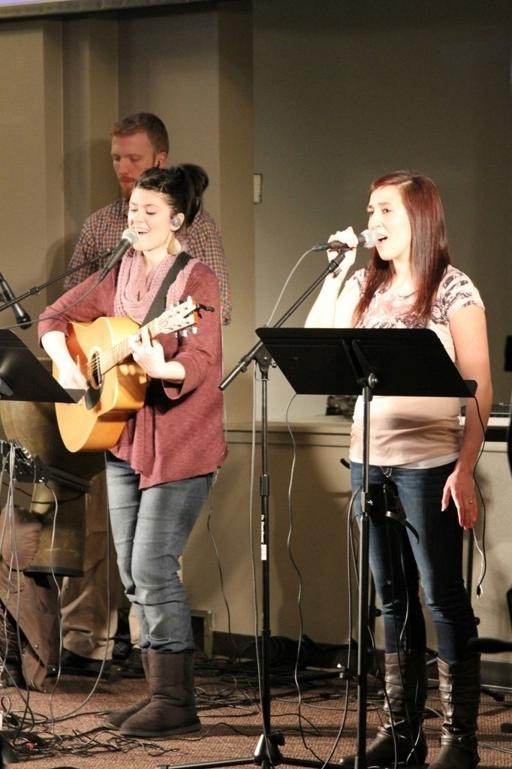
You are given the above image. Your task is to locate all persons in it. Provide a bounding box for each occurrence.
[40,162,216,738]
[53,111,231,671]
[305,169,493,768]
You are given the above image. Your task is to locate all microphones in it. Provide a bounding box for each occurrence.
[0,277,31,328]
[320,228,379,249]
[99,227,136,280]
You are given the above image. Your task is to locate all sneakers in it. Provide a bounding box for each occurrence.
[48,647,142,680]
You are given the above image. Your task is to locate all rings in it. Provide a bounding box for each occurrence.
[469,500,474,504]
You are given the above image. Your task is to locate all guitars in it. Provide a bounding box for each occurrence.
[53,295,215,454]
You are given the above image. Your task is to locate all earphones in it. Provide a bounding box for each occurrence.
[172,218,180,226]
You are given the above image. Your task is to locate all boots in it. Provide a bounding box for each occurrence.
[120,649,203,738]
[340,652,429,769]
[108,647,149,729]
[428,657,481,768]
